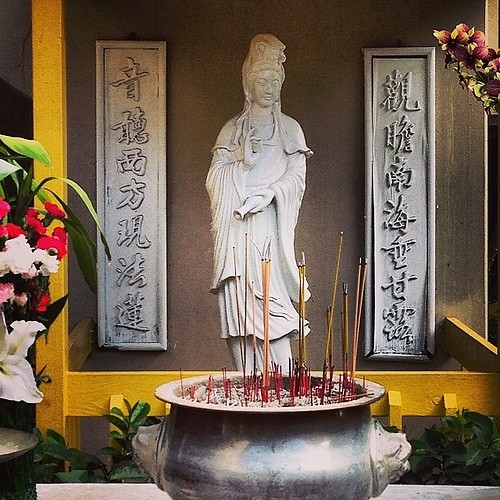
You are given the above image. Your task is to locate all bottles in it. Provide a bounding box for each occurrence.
[234,197,261,220]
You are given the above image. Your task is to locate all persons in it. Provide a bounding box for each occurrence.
[205,34,314,373]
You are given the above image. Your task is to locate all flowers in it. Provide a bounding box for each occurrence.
[0,135,112,431]
[433,23,500,115]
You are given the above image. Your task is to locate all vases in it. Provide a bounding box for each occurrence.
[0,399,33,500]
